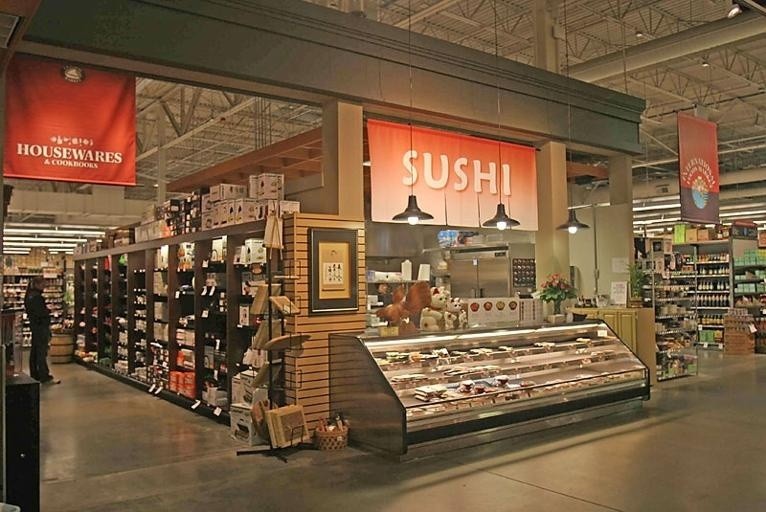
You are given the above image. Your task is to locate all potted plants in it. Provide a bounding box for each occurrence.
[626,264,649,307]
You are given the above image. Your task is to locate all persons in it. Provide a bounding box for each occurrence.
[371,280,391,307]
[23,276,61,385]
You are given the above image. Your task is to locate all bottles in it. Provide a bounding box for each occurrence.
[696,252,730,325]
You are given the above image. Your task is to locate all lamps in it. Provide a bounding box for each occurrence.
[393,0,434,225]
[556,0,591,234]
[482,3,521,230]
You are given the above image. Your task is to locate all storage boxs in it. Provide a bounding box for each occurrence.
[673,223,716,243]
[724,334,755,355]
[200,171,285,232]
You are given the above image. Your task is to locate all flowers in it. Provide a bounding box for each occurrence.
[539,273,579,303]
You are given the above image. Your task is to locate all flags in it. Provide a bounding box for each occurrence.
[1,57,138,188]
[676,112,722,225]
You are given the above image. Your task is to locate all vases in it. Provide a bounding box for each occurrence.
[553,302,561,315]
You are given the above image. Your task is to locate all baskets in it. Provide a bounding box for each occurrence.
[315,426,350,451]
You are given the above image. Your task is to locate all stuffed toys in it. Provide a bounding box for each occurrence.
[443,297,469,331]
[418,286,457,331]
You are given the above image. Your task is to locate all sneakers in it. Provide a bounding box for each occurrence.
[42,378,60,384]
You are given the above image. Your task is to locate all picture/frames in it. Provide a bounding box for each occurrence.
[306,226,361,314]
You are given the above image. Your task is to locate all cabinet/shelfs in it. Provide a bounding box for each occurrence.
[567,306,657,387]
[643,243,699,382]
[3,272,65,349]
[71,220,318,462]
[672,237,766,346]
[327,319,651,462]
